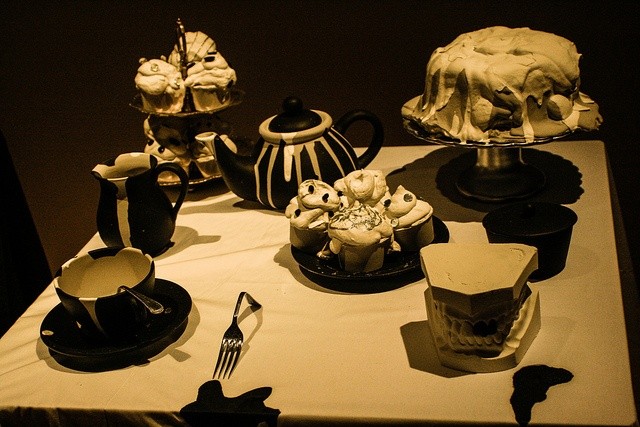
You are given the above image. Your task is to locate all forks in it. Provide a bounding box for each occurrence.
[212,292,262,379]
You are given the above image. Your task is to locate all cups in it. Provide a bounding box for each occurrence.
[91,152,188,254]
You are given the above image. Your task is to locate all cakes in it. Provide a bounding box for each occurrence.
[285,169,435,275]
[135,31,237,182]
[402,26,603,143]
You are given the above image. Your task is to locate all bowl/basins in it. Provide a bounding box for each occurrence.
[55,245,157,334]
[482,201,578,283]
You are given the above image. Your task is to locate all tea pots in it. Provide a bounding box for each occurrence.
[195,101,386,211]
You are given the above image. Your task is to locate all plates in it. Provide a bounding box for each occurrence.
[39,277,193,371]
[283,216,449,291]
